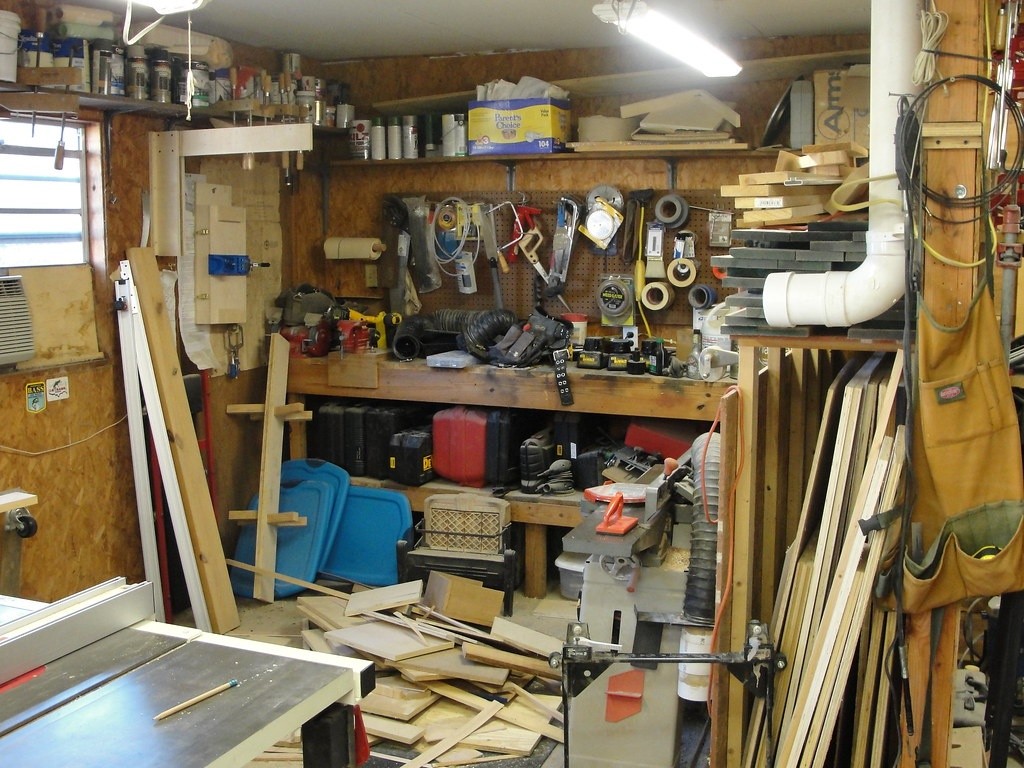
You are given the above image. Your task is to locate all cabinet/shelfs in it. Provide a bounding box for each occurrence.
[283,348,789,631]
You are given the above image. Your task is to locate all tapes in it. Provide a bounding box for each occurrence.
[667,257,698,287]
[640,281,676,311]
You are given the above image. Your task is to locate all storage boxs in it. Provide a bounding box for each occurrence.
[468,97,572,155]
[552,555,590,601]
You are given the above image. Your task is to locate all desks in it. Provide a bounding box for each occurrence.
[0,591,376,768]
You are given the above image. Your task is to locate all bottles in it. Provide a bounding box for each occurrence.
[371,115,418,160]
[299,90,318,123]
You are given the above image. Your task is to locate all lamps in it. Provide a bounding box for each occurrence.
[593,0,742,79]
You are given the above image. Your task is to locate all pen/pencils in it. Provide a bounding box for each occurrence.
[152,678,241,723]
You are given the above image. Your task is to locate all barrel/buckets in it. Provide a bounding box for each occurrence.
[0,9,24,82]
